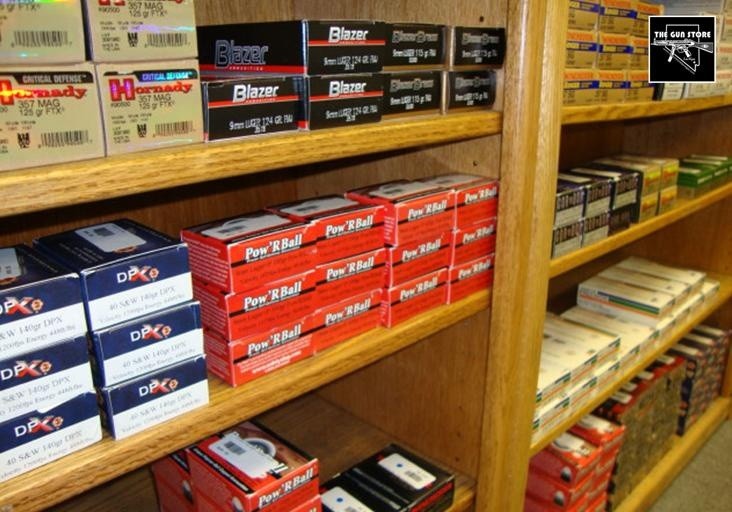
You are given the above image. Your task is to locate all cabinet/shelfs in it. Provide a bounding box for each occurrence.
[3,0,545,186]
[532,105,732,275]
[2,109,516,512]
[542,2,728,121]
[1,284,513,512]
[503,282,729,511]
[510,185,730,456]
